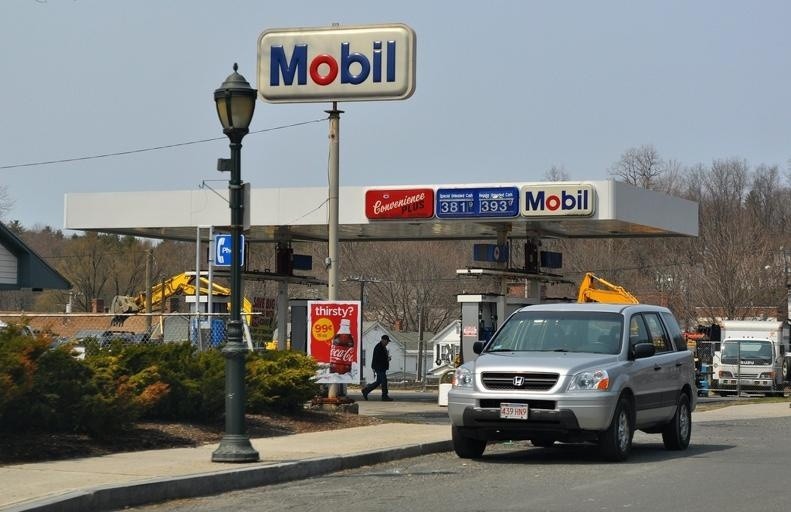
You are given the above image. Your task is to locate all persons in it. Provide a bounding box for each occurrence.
[361,335,393,401]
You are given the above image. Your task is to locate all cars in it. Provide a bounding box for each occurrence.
[379,370,424,385]
[0,320,147,363]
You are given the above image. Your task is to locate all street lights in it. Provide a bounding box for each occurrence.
[212,59,258,464]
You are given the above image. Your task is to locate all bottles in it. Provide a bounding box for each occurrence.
[329,318,355,375]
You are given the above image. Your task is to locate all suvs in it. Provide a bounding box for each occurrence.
[450,303,698,461]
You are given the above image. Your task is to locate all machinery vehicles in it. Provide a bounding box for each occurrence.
[108,270,290,355]
[577,271,710,397]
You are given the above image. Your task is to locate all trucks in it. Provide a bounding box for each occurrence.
[712,316,791,398]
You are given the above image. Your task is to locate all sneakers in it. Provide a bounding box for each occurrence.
[361,389,369,401]
[381,395,394,401]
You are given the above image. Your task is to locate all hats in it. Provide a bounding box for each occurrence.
[382,335,392,342]
